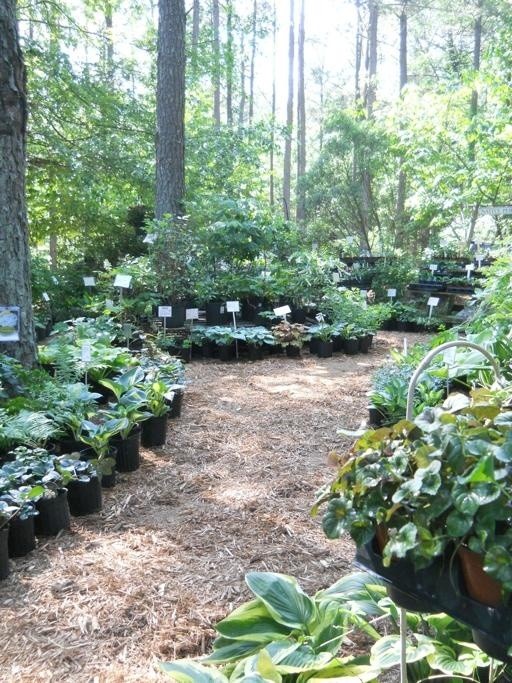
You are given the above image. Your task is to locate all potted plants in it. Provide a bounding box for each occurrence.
[0,333,190,581]
[158,247,384,364]
[307,248,510,662]
